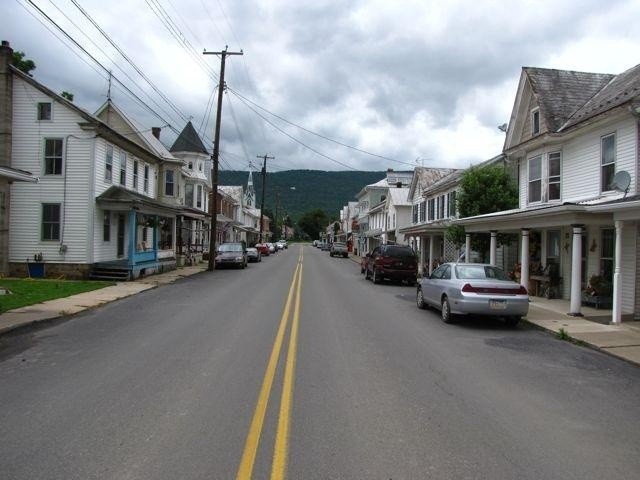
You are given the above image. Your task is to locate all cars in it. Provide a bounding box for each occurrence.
[215,239,287,269]
[317,242,349,257]
[417,262,529,327]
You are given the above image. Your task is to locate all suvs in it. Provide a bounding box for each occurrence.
[361,245,418,287]
